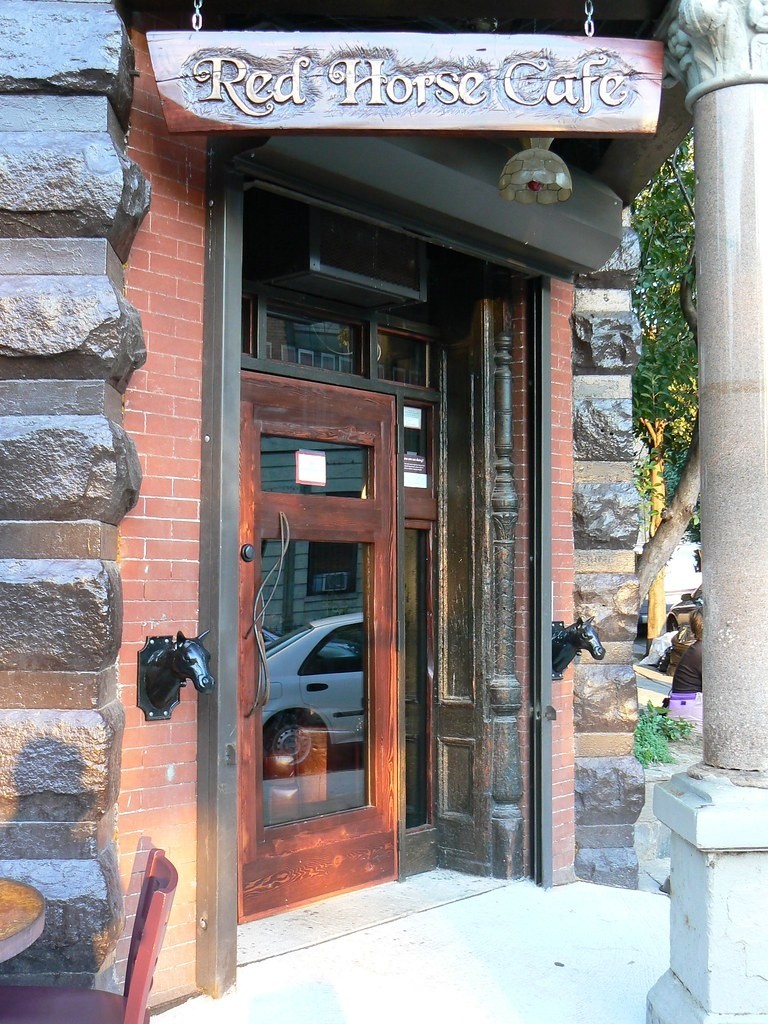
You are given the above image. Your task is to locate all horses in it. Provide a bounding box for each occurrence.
[548,615,606,673]
[146,630,216,710]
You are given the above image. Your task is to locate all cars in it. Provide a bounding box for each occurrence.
[263,612,367,773]
[666,583,702,633]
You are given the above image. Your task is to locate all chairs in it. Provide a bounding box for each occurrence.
[0,848,178,1024]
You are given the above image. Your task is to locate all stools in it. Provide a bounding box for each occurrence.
[668,690,697,717]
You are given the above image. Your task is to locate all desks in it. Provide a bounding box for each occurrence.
[0,876,45,963]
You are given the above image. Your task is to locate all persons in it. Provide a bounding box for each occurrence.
[669,604,702,694]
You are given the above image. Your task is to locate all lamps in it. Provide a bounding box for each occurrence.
[500,137,573,205]
